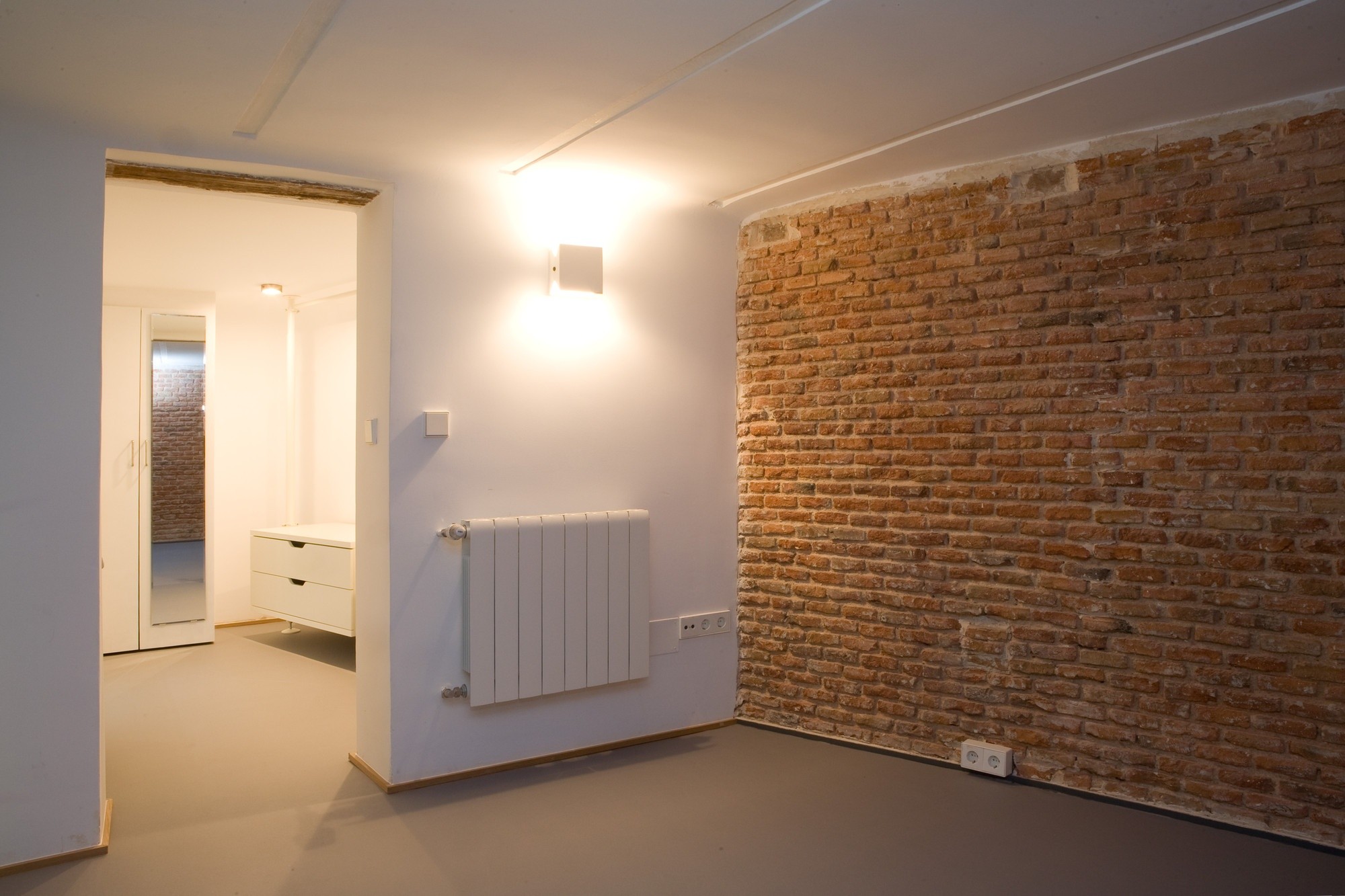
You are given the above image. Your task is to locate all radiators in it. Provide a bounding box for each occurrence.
[437,509,650,708]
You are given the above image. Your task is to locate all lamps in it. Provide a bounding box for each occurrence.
[260,284,283,295]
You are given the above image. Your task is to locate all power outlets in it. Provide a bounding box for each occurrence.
[960,739,1013,778]
[697,609,730,638]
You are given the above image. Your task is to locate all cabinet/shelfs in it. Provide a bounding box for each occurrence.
[248,522,356,639]
